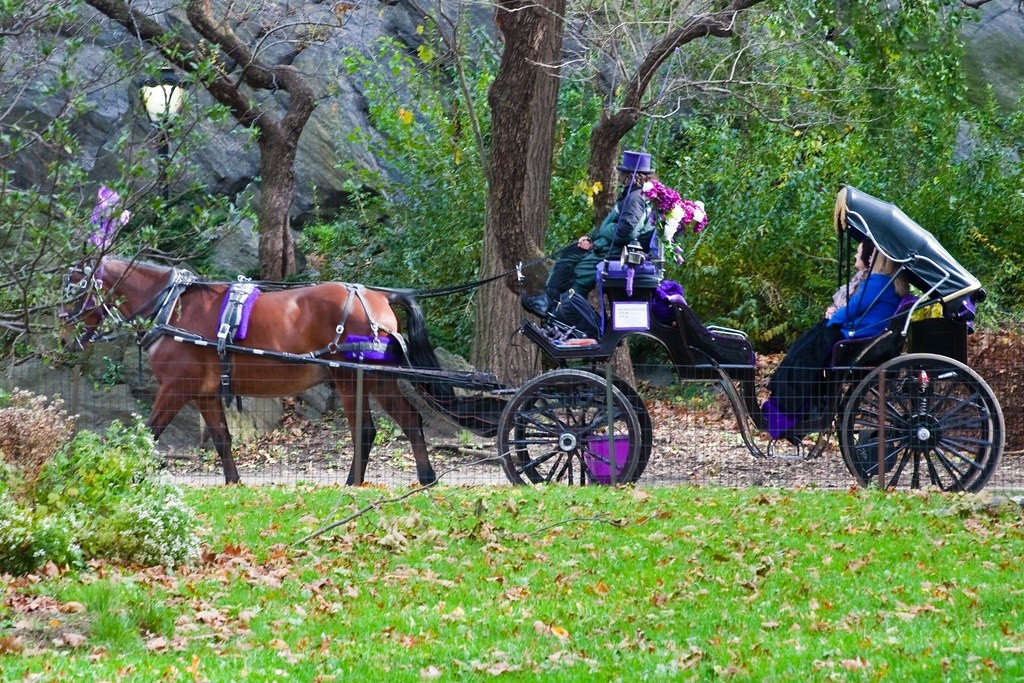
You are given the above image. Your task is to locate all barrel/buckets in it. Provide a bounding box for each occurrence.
[586,436,629,484]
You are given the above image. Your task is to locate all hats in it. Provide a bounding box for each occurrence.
[616,151,654,174]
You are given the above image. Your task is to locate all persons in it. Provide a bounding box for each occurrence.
[761,238,911,416]
[522,151,657,318]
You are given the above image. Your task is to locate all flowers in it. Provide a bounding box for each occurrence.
[640,178,709,266]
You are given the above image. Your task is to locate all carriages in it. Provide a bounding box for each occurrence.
[57,186,1009,504]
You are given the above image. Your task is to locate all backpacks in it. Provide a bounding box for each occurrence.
[546,290,604,344]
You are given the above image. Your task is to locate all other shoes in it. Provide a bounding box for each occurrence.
[520,288,551,319]
[801,400,829,422]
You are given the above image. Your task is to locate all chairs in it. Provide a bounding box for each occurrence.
[598,229,659,286]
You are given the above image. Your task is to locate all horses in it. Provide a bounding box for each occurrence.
[57,240,457,489]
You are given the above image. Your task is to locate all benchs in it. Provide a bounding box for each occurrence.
[831,295,918,371]
[653,282,757,377]
